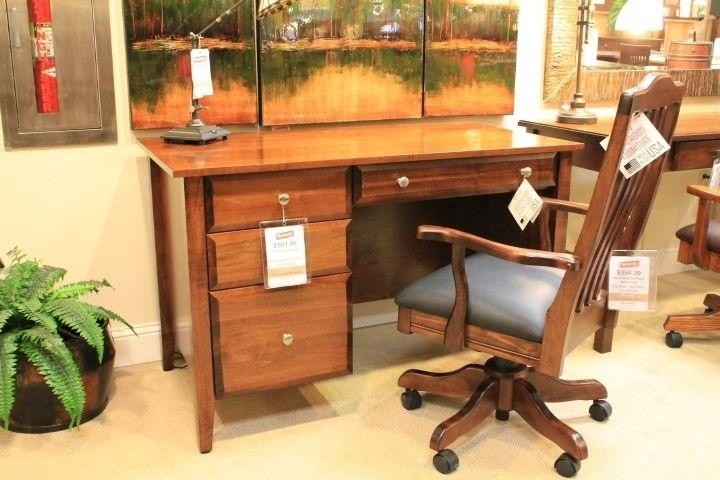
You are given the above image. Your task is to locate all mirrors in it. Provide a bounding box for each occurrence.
[540,0,720,105]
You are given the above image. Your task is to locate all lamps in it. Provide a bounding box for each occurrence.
[157,0,300,144]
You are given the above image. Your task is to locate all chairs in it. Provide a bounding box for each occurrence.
[617,41,651,66]
[393,70,684,480]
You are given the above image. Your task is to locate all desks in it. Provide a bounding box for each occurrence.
[127,105,584,455]
[519,98,719,173]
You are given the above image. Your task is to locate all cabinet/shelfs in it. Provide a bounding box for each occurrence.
[196,168,359,401]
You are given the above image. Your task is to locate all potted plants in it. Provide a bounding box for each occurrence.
[0,246,140,436]
